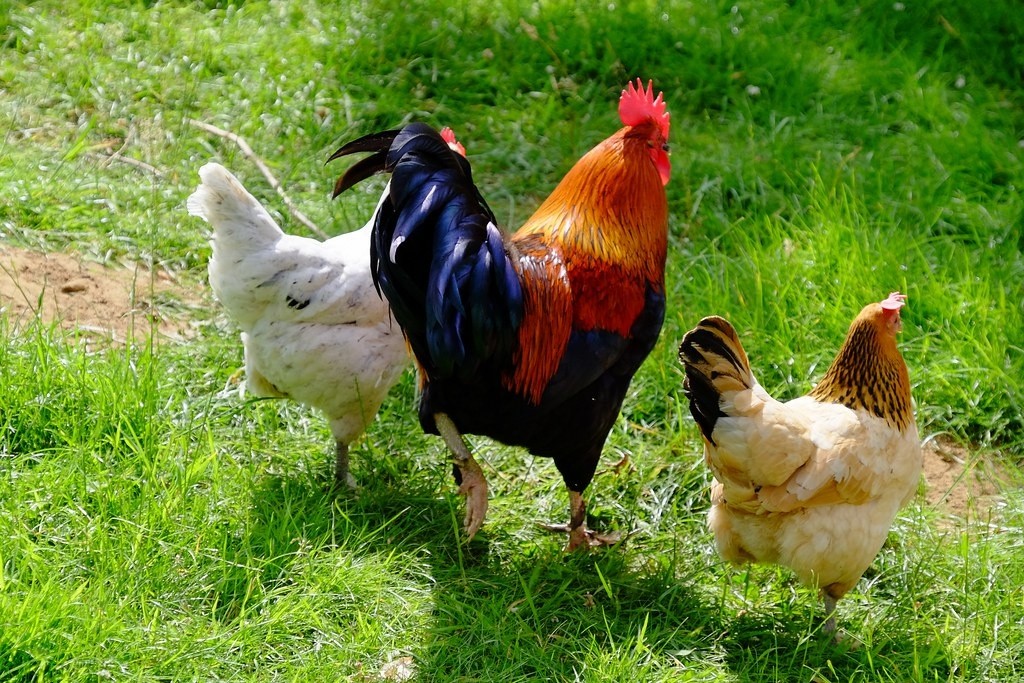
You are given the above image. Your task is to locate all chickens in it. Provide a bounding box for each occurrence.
[184,128,465,501]
[678,290,923,644]
[326,77,672,558]
